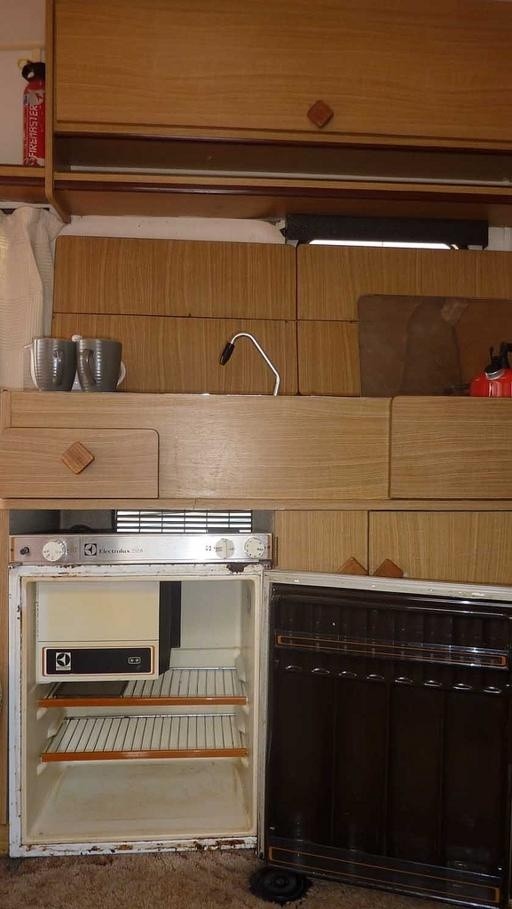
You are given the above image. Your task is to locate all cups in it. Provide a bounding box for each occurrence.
[76,337,124,392]
[28,337,80,392]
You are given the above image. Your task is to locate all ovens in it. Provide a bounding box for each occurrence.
[6,524,512,908]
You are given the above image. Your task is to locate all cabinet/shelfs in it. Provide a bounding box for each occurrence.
[11,401,512,585]
[56,0,512,200]
